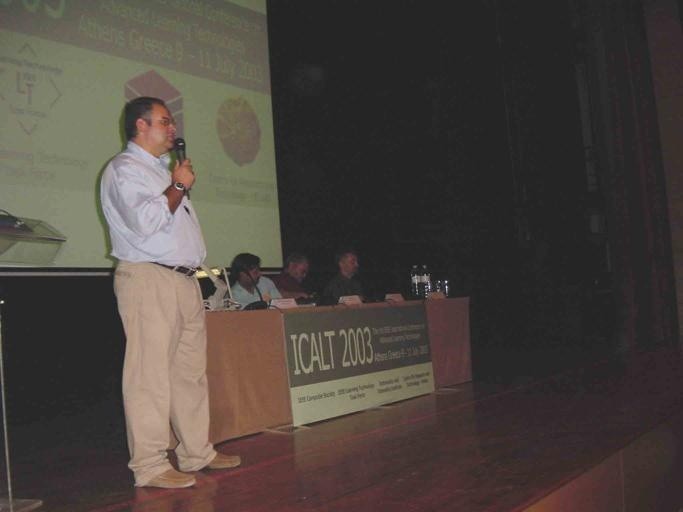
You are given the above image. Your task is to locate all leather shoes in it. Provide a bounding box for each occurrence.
[206,450,242,469]
[144,467,196,490]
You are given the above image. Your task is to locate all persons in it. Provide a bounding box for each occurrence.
[224,253,283,312]
[321,250,386,305]
[99,97,242,492]
[276,242,321,303]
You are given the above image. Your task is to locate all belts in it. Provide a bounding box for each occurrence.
[145,262,199,278]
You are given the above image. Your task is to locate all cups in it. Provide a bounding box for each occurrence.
[434,280,449,298]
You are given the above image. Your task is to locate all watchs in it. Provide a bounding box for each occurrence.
[172,180,190,200]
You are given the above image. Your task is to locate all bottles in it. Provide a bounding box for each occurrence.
[410,264,432,299]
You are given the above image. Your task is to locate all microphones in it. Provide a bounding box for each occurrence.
[237,268,267,309]
[175,138,189,200]
